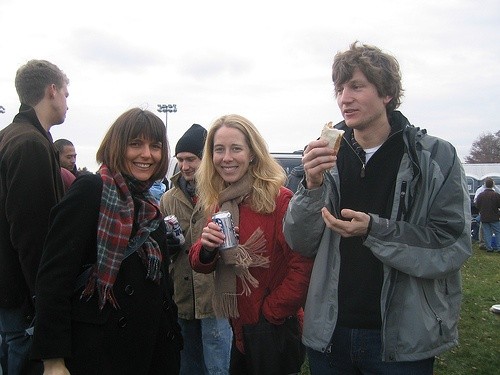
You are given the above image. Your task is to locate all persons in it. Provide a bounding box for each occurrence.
[0,59,69,375]
[158,122,233,375]
[473,176,500,250]
[474,180,500,253]
[53,139,85,179]
[29,107,185,375]
[187,113,316,375]
[283,40,471,375]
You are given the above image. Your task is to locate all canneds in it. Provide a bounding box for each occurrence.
[211,210,238,251]
[163,215,185,245]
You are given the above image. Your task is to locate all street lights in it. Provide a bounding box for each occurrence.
[157,103,177,129]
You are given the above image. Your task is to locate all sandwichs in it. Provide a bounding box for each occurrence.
[319,121,345,156]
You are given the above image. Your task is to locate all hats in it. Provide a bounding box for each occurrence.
[175,124,208,160]
